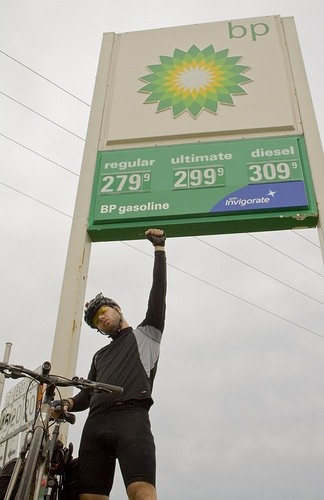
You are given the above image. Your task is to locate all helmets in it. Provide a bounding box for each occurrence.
[84,292,121,329]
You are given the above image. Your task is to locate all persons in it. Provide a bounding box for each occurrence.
[50,229,168,500]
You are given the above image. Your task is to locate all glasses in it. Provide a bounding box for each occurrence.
[92,304,109,327]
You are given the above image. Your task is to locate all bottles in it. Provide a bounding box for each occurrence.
[44,472,58,500]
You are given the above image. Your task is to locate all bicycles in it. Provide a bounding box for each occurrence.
[1,360,124,500]
